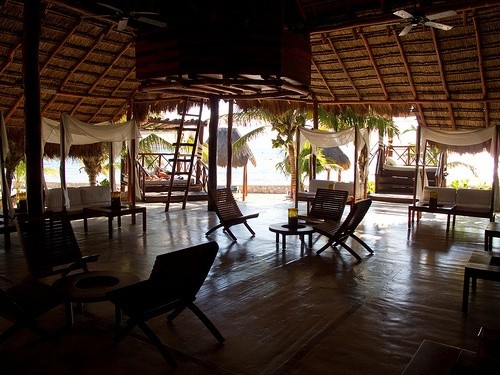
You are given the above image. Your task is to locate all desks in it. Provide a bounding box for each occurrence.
[484,222,500,252]
[51,271,140,327]
[83,205,146,238]
[407,200,457,233]
[269,222,314,251]
[462,249,500,312]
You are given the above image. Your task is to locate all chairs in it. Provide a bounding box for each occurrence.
[107,241,226,369]
[299,188,373,261]
[401,325,500,375]
[205,188,259,241]
[0,214,100,351]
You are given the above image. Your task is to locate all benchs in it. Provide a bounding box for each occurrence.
[295,180,362,210]
[142,169,210,202]
[45,186,110,240]
[365,166,441,204]
[417,187,496,228]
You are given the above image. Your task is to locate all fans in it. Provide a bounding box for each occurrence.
[370,0,458,37]
[81,0,167,32]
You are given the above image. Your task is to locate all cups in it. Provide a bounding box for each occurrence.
[110,192,121,210]
[429,192,437,209]
